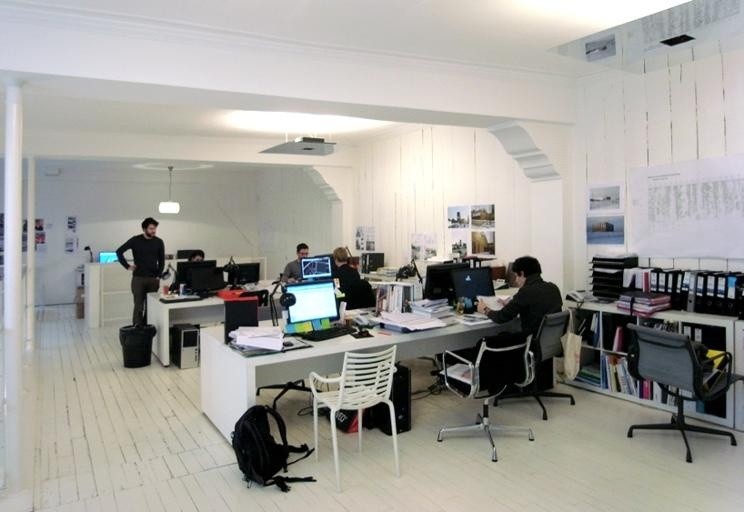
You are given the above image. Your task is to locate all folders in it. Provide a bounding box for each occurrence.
[650,268,744,317]
[683,325,727,361]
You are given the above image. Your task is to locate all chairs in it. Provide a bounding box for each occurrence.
[309,345,400,493]
[362,285,387,316]
[493,310,575,419]
[626,323,744,462]
[437,331,534,462]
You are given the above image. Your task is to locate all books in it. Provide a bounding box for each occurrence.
[337,267,514,333]
[572,312,705,420]
[615,290,672,319]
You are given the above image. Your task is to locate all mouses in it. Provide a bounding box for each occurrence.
[359,330,369,337]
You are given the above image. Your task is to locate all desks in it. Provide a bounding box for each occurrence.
[200,305,522,445]
[157,256,267,287]
[147,281,286,367]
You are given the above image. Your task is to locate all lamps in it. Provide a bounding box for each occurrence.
[84,246,93,263]
[159,166,180,214]
[392,259,425,299]
[222,256,246,290]
[269,281,296,327]
[152,263,186,290]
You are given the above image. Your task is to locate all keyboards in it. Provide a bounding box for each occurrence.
[302,325,357,341]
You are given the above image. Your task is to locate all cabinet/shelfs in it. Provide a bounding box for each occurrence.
[85,259,134,328]
[562,300,744,430]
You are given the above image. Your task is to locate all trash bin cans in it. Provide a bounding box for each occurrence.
[120,324,155,368]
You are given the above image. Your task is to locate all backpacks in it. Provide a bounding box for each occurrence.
[231,404,317,491]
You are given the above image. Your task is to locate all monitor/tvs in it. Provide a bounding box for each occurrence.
[228,263,259,290]
[300,256,333,281]
[98,251,119,264]
[450,266,495,314]
[176,249,224,297]
[282,279,339,331]
[423,263,470,306]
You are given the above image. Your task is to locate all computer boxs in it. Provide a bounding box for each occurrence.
[172,323,199,369]
[376,364,411,436]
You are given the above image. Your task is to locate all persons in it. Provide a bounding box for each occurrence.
[331,247,361,294]
[115,217,164,326]
[476,255,563,364]
[280,242,309,284]
[187,249,205,261]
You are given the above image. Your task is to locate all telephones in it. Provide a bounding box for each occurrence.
[354,312,376,328]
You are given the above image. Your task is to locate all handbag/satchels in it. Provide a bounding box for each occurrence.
[560,308,582,382]
